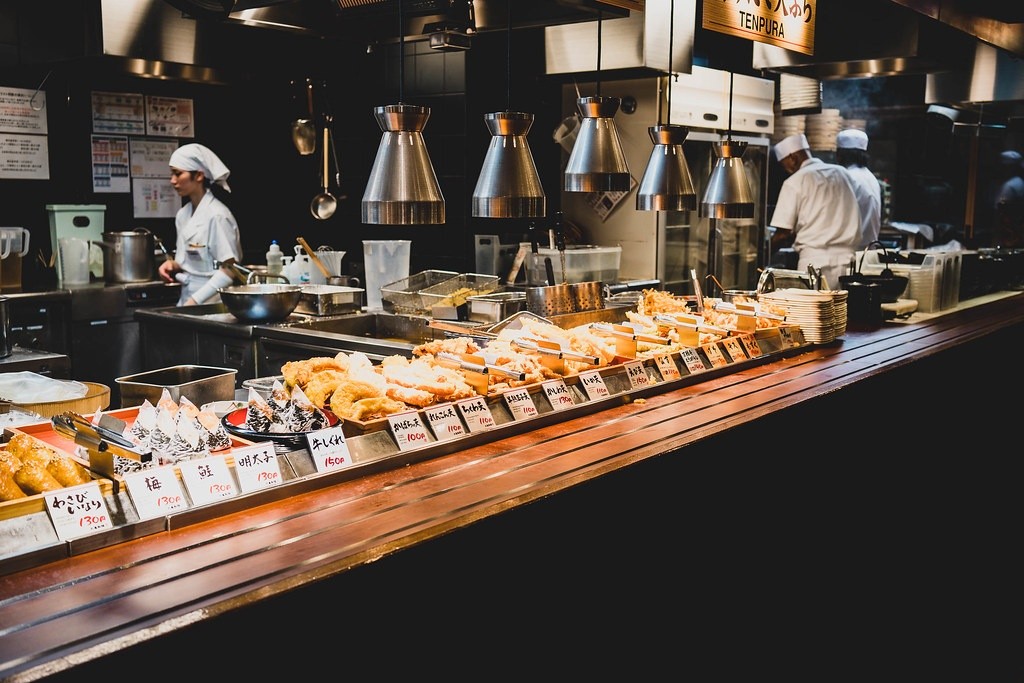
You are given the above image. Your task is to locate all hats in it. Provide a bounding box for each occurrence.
[774,133,809,161]
[837,130,868,151]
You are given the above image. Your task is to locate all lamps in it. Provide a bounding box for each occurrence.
[469,0,548,219]
[700,68,756,220]
[635,0,698,213]
[362,0,447,225]
[565,6,631,192]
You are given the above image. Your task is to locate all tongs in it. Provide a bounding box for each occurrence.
[588,315,732,346]
[689,268,705,312]
[808,263,820,289]
[51,411,135,448]
[435,336,601,381]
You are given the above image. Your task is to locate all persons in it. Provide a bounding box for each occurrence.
[159,143,243,307]
[993,150,1024,232]
[837,129,882,250]
[765,134,864,289]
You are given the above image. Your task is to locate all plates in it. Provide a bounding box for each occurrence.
[221,405,341,454]
[199,400,250,418]
[755,287,848,345]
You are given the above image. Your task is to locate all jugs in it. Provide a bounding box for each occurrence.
[56,237,90,291]
[0,226,29,295]
[308,252,346,285]
[362,240,412,313]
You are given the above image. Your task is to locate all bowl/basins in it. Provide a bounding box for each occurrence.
[215,284,305,324]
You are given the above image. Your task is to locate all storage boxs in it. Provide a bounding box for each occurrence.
[522,243,625,286]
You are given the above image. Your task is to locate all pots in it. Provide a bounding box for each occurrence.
[766,267,816,290]
[93,231,155,283]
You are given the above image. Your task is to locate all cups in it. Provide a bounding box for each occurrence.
[327,277,361,289]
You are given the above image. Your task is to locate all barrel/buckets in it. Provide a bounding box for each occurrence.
[843,120,867,133]
[723,290,757,304]
[771,109,841,151]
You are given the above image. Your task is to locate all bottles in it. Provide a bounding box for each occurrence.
[266,240,284,284]
